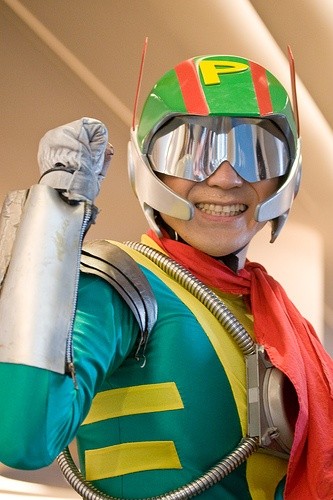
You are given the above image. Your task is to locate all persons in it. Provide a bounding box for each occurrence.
[0,54,333,500]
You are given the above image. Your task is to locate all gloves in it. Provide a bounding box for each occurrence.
[37,117,114,201]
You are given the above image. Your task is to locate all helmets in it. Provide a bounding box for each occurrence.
[127,56,303,243]
